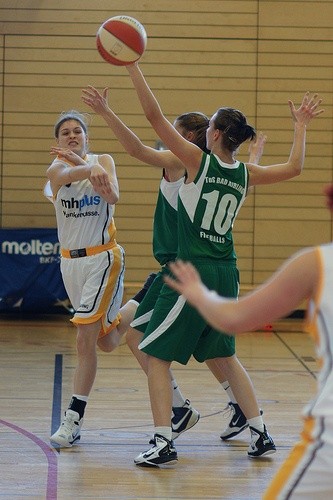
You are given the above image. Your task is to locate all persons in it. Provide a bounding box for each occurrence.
[42,109,162,446]
[80,63,326,465]
[162,186,333,500]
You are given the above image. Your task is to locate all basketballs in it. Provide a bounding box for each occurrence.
[97,17,147,66]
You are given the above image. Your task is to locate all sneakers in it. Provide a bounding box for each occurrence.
[50,408,87,447]
[219,400,264,440]
[133,432,178,466]
[171,397,201,441]
[246,424,277,458]
